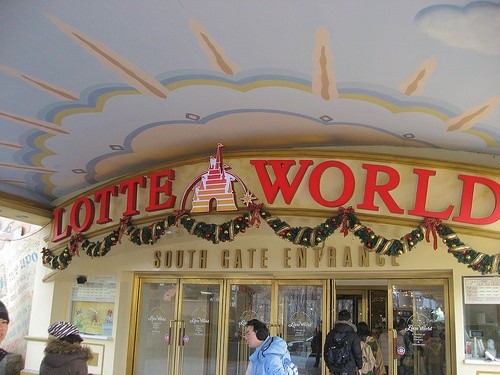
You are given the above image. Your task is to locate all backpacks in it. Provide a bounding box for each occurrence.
[359,336,377,374]
[325,328,352,368]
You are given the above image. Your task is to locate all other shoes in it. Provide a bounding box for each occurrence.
[313,365,319,368]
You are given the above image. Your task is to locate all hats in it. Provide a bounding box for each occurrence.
[0,300,9,324]
[48,321,80,340]
[338,310,351,321]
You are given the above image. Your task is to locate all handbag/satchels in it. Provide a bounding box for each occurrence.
[401,356,414,367]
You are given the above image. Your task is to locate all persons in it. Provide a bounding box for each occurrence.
[0,300,25,375]
[242,319,300,375]
[310,308,447,375]
[39,321,93,375]
